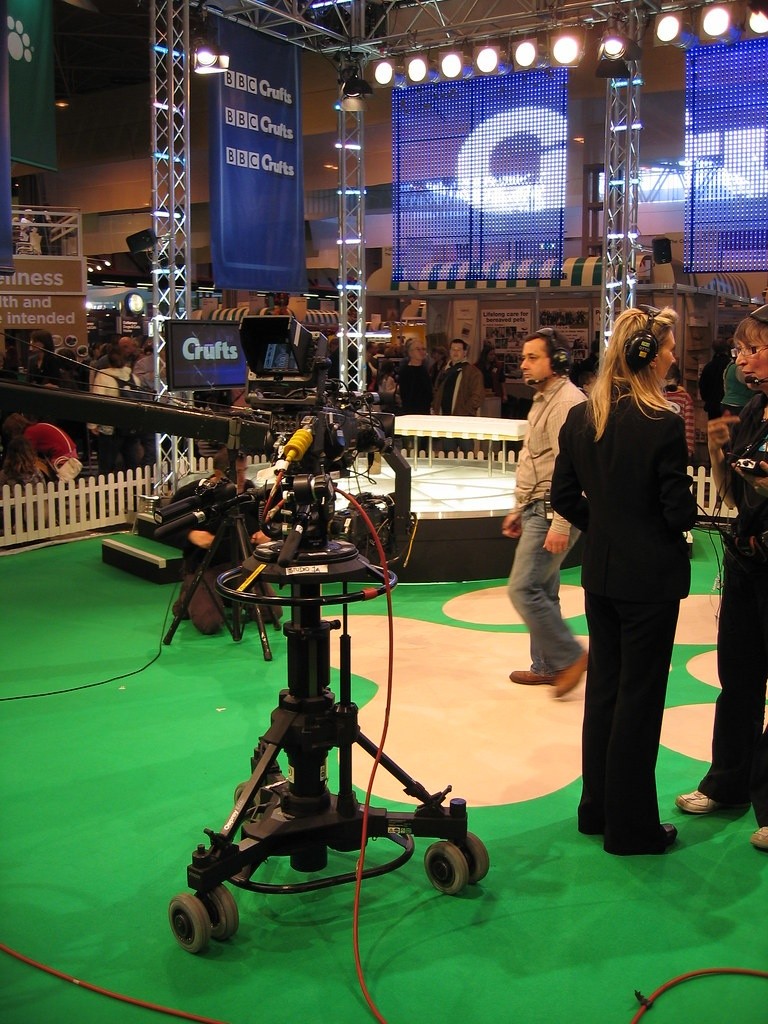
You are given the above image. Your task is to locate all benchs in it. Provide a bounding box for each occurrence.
[395,414,527,477]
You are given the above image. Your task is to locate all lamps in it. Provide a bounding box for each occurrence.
[126,228,158,254]
[337,66,374,112]
[369,0,768,88]
[189,36,231,74]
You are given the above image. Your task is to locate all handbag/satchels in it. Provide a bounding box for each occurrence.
[54,455,83,483]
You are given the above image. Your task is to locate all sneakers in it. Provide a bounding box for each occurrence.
[675,790,751,814]
[750,827,768,847]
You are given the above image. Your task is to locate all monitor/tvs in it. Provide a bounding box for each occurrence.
[166,319,249,391]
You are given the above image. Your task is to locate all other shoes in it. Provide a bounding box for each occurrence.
[662,823,677,844]
[510,670,559,686]
[554,651,589,698]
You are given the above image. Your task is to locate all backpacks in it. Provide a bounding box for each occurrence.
[112,373,145,437]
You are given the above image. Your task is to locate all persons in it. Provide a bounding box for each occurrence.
[474,341,505,397]
[330,336,448,458]
[13,208,37,242]
[0,412,80,497]
[550,303,700,854]
[502,331,590,700]
[430,338,486,459]
[0,330,170,511]
[674,307,768,853]
[0,436,53,531]
[171,448,283,635]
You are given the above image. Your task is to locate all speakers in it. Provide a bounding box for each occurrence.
[652,236,672,264]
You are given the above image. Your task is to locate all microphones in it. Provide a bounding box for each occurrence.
[744,376,768,386]
[364,392,396,405]
[528,372,558,385]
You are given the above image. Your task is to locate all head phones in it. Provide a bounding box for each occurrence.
[536,327,570,374]
[626,302,660,373]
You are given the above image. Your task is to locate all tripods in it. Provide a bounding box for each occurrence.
[162,448,281,662]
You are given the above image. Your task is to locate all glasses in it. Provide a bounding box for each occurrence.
[730,345,768,357]
